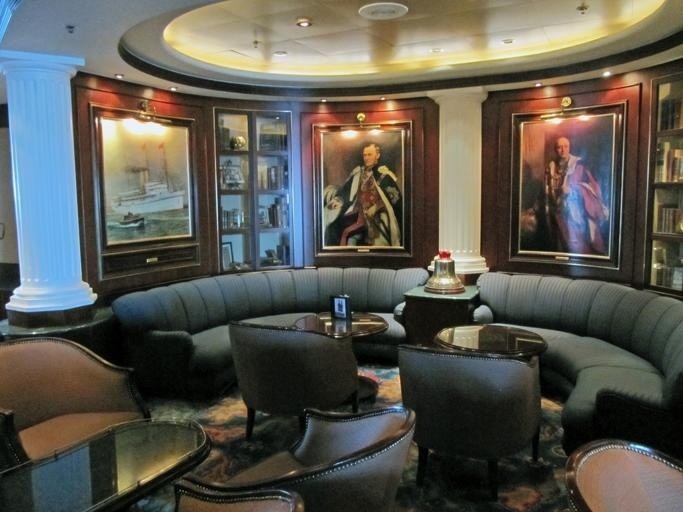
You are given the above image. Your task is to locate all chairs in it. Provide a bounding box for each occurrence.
[223,318,359,444]
[167,403,418,510]
[1,336,152,512]
[0,414,215,511]
[165,482,305,512]
[396,344,540,500]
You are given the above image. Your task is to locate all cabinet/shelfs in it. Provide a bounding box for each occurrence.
[646,69,683,302]
[210,100,303,270]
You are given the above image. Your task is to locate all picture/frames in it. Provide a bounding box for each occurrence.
[87,103,201,259]
[311,119,414,260]
[220,165,245,186]
[508,101,630,271]
[221,241,235,269]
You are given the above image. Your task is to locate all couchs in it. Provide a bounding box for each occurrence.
[469,271,681,457]
[112,267,428,407]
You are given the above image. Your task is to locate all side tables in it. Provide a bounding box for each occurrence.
[404,282,482,353]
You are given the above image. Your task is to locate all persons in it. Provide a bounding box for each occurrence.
[540,137,609,256]
[329,143,401,246]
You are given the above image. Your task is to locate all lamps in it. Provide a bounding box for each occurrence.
[356,3,409,22]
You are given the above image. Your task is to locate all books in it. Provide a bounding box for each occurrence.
[660,206,681,233]
[655,141,683,182]
[663,98,683,129]
